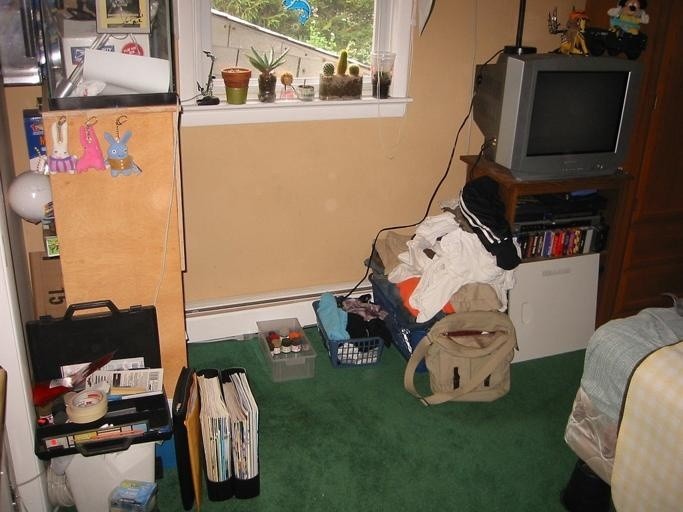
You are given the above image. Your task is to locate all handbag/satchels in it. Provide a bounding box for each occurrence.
[403,304,521,405]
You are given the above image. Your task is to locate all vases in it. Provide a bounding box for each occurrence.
[298,86,314,101]
[220,67,252,104]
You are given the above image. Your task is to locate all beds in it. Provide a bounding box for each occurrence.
[559,303,682,511]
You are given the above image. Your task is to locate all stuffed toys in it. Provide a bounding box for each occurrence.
[74,125,105,174]
[41,120,78,176]
[102,131,140,177]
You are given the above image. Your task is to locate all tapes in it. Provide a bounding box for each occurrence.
[65,389,108,424]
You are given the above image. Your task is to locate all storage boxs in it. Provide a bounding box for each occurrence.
[59,16,150,90]
[255,317,317,382]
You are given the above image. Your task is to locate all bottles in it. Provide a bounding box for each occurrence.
[264,327,301,356]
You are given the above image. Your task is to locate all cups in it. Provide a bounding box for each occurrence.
[298,85,314,99]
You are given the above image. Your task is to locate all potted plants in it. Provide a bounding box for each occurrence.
[247,43,289,103]
[317,49,362,100]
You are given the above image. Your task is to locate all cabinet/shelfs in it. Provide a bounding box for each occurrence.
[459,153,635,265]
[46,99,189,402]
[582,2,683,330]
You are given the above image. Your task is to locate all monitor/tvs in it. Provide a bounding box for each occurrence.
[472,52,645,184]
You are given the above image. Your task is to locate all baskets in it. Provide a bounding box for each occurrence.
[311,301,384,367]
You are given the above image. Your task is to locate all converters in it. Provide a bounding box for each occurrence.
[504,45,537,55]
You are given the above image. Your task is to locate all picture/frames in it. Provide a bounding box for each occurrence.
[94,1,151,35]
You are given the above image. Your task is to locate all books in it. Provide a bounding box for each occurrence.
[517,226,599,258]
[181,366,260,502]
